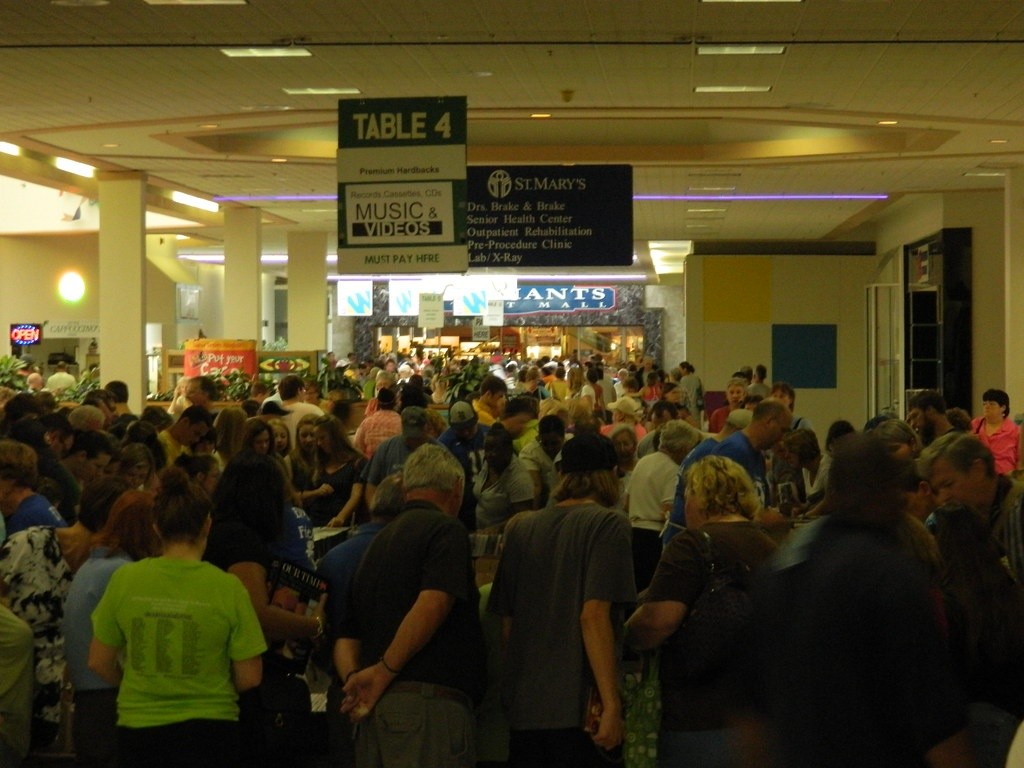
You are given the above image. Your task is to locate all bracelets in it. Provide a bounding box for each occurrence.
[313,615,324,639]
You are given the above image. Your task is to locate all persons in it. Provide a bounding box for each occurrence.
[280,415,329,492]
[172,452,222,495]
[785,440,975,768]
[971,389,1020,472]
[301,413,367,528]
[118,443,153,489]
[63,490,158,766]
[625,455,785,768]
[317,472,401,768]
[0,439,67,537]
[1,350,970,463]
[488,430,637,768]
[472,422,538,531]
[935,496,1024,768]
[336,442,489,768]
[711,397,793,507]
[0,602,34,767]
[40,431,114,525]
[0,477,133,768]
[90,471,269,768]
[624,420,702,592]
[915,432,1023,589]
[203,452,329,766]
[661,409,755,559]
[784,428,836,518]
[364,407,449,522]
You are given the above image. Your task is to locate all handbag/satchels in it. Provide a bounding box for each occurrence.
[619,645,665,768]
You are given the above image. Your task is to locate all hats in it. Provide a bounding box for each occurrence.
[554,432,626,478]
[400,406,427,439]
[448,399,478,430]
[606,396,643,416]
[261,401,290,416]
[726,409,754,428]
